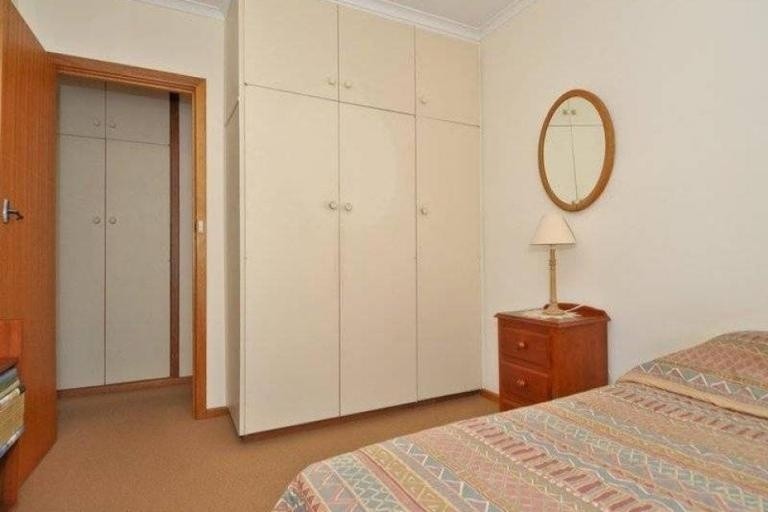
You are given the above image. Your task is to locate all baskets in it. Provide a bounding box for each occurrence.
[0,387,26,449]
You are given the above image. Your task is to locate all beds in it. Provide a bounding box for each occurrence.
[270,328,768,512]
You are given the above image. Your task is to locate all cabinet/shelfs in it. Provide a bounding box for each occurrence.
[416,114,482,403]
[223,0,415,125]
[223,84,417,438]
[59,73,171,145]
[55,135,173,392]
[543,96,606,204]
[415,26,482,127]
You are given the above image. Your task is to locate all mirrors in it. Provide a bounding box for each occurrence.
[537,88,616,213]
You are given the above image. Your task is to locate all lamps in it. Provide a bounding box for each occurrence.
[528,214,577,317]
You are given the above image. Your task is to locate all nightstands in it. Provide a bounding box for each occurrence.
[493,302,611,412]
